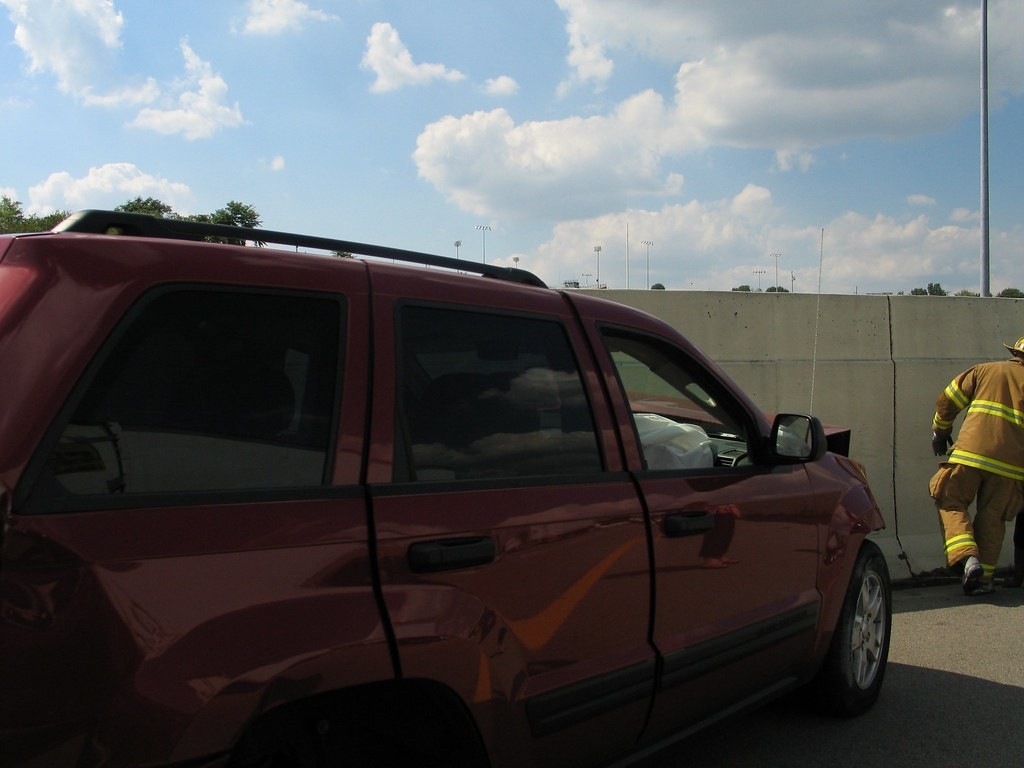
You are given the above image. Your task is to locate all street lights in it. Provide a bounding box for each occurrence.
[640,241,654,289]
[594,246,601,289]
[770,253,782,292]
[513,257,519,268]
[752,271,766,292]
[582,274,593,288]
[475,226,492,264]
[454,241,462,274]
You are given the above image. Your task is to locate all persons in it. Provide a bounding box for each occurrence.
[928,337,1024,596]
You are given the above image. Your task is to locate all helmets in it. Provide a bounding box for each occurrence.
[1002,337,1024,357]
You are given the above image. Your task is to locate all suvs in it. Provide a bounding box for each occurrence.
[0,210,894,768]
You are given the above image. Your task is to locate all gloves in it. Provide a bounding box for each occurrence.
[932,432,953,456]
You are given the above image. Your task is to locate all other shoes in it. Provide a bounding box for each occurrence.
[966,578,995,596]
[1002,574,1024,587]
[960,557,983,592]
